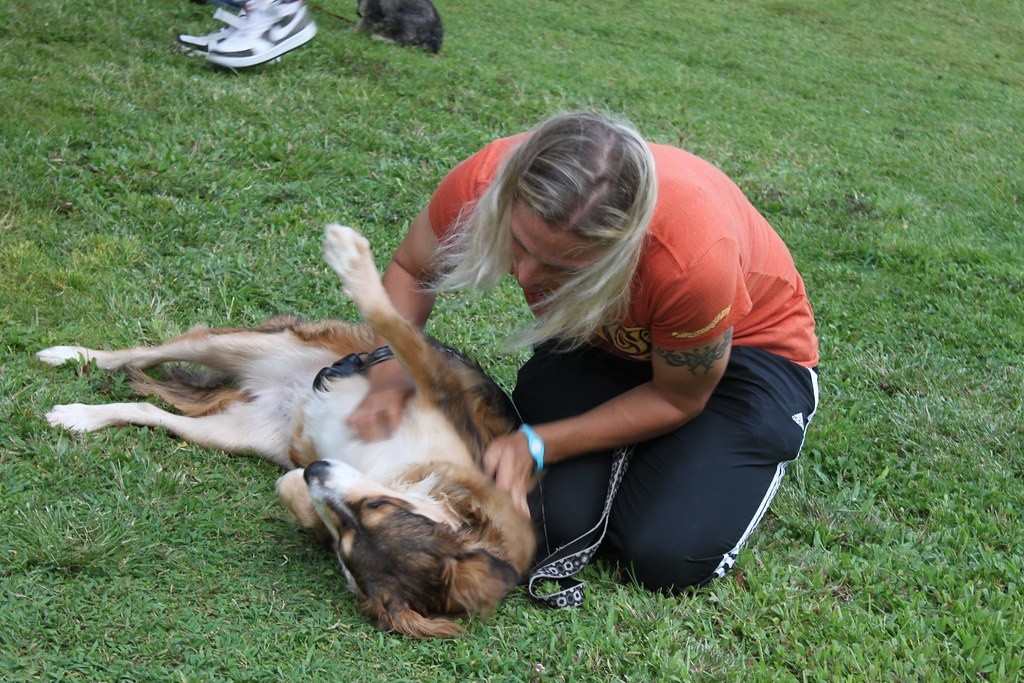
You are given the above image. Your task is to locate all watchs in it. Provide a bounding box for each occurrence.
[519,422,545,472]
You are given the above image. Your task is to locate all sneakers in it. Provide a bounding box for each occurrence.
[206,0,319,67]
[178,7,245,54]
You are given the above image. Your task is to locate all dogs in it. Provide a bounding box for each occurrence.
[32,220,548,642]
[340,0,443,57]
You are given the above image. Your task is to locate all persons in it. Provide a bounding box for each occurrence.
[176,0,318,70]
[344,111,820,593]
